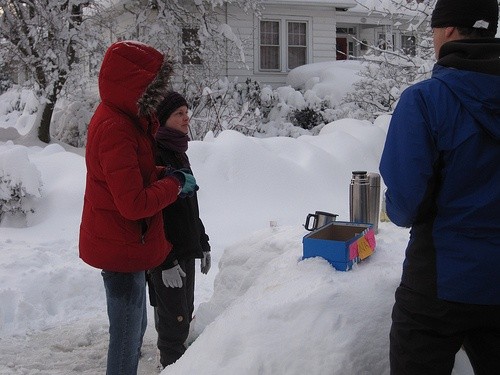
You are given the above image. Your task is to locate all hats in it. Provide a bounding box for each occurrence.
[431,0,498,33]
[158,91,189,127]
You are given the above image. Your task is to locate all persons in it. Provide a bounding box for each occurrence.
[79,41,199,375]
[379,0,500,375]
[146,91,212,369]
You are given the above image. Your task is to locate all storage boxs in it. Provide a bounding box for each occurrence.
[302,221,376,272]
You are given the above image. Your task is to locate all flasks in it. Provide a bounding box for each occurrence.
[349,171,369,224]
[369,173,380,233]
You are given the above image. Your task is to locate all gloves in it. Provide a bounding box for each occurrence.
[162,264,186,288]
[172,170,196,194]
[169,167,199,199]
[201,251,212,275]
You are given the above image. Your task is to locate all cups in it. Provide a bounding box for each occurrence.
[305,211,339,231]
